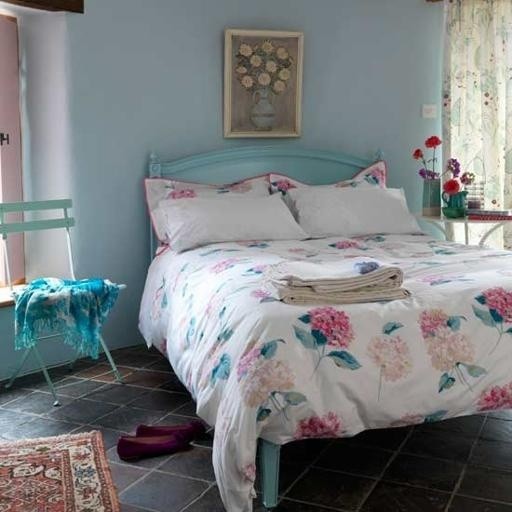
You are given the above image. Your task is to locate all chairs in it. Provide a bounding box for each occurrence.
[0,198,127,406]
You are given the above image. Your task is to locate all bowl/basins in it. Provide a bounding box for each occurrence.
[442,206,465,219]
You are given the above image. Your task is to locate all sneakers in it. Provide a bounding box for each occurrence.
[136,418,206,442]
[118,431,189,461]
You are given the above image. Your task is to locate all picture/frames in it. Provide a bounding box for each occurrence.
[223,28,304,139]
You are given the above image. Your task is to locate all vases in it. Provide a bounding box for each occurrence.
[248,88,277,131]
[422,179,441,216]
[441,191,468,218]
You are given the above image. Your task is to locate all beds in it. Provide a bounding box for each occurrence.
[136,144,512,512]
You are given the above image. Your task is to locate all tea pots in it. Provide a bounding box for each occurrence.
[441,190,469,209]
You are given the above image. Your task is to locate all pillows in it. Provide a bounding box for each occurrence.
[144,161,425,255]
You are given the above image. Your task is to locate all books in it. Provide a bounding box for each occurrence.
[464,209,511,220]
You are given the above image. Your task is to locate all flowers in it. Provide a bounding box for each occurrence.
[410,136,461,180]
[231,39,294,93]
[443,172,477,195]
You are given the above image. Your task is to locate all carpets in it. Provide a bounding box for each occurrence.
[0,430,121,512]
